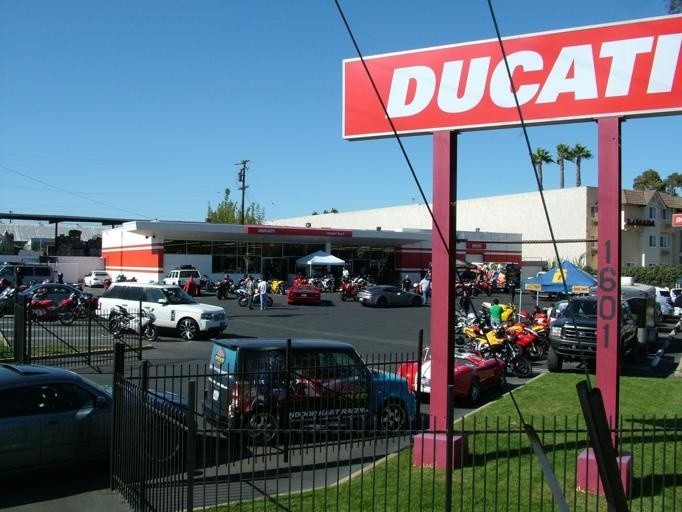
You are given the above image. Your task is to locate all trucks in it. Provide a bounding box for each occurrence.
[417,262,522,299]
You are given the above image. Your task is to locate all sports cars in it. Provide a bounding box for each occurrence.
[356,284,425,310]
[287,285,322,307]
[393,342,508,406]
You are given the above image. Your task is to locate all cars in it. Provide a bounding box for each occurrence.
[198,336,417,442]
[0,360,191,488]
[80,270,111,288]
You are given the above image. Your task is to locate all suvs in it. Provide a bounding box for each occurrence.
[162,268,203,293]
[0,263,57,289]
[93,282,228,341]
[547,285,682,375]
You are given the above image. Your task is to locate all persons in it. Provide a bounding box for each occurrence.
[17,267,25,286]
[257,278,270,310]
[508,279,519,303]
[341,266,350,292]
[459,291,470,315]
[247,275,255,310]
[403,274,411,292]
[418,275,431,304]
[490,298,504,330]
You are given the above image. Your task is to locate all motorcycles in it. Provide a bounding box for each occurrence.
[0,282,100,325]
[105,304,162,344]
[207,276,284,308]
[115,273,138,283]
[303,272,370,304]
[453,303,551,380]
